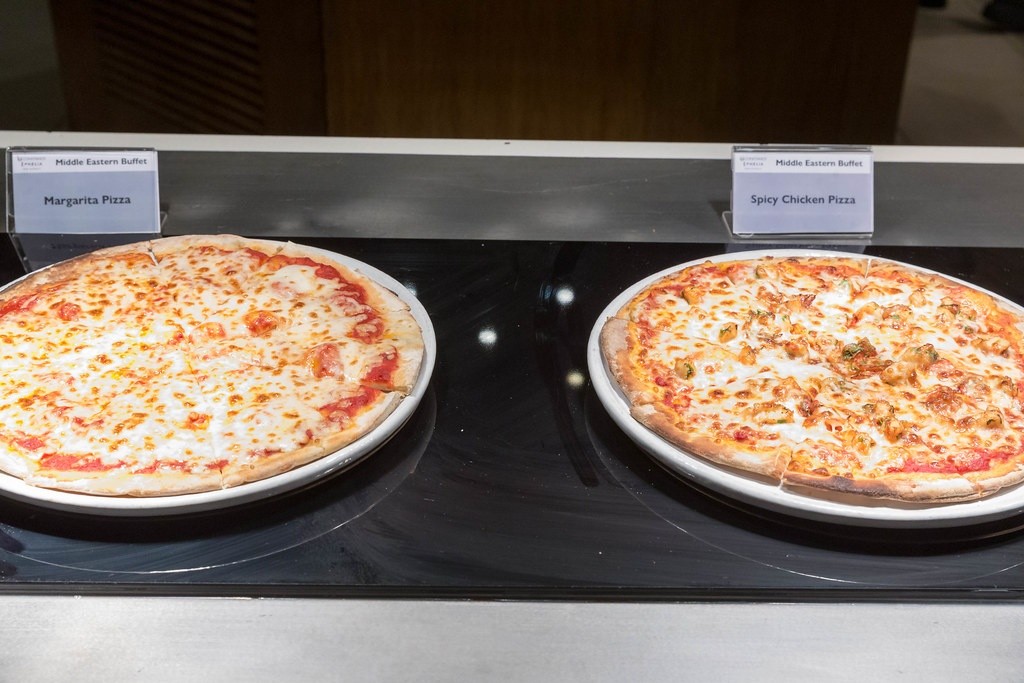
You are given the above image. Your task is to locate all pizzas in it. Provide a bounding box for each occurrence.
[0,233,425,498]
[599,256,1024,504]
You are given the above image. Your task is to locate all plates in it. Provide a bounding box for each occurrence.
[587,248,1024,526]
[0,239,437,514]
